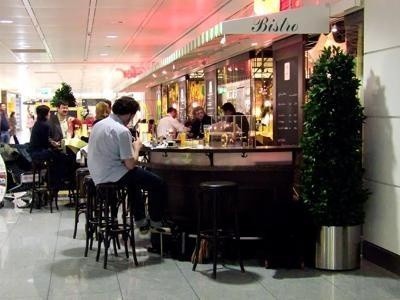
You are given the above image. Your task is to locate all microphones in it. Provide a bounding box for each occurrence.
[193,181,246,278]
[73,166,109,241]
[96,182,139,270]
[31,158,60,215]
[83,175,121,257]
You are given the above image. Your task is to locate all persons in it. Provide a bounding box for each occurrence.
[87,97,178,235]
[50,103,80,160]
[92,102,110,127]
[30,112,35,120]
[221,102,250,143]
[157,107,190,140]
[188,106,216,140]
[8,112,21,146]
[30,105,69,196]
[27,114,35,134]
[0,103,12,146]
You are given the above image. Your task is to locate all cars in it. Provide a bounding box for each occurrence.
[203,124,210,143]
[180,127,187,146]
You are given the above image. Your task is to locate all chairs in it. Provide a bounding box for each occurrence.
[139,224,151,234]
[149,225,172,234]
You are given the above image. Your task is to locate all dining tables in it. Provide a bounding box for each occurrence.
[298,46,369,272]
